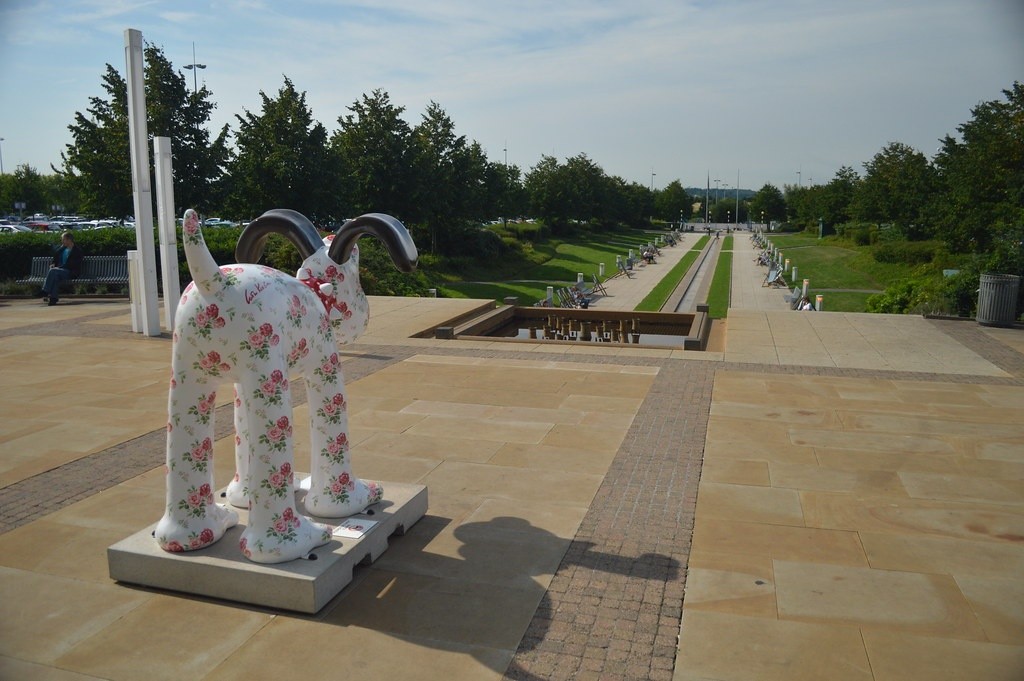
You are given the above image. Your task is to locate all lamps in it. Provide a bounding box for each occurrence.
[598,262,605,276]
[546,286,554,303]
[577,272,584,283]
[815,295,824,311]
[640,244,643,250]
[655,238,659,247]
[784,259,791,272]
[647,242,651,247]
[802,279,809,297]
[760,232,783,265]
[616,255,622,267]
[792,266,798,281]
[628,249,633,258]
[660,229,678,242]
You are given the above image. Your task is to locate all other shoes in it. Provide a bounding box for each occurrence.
[48,298,58,306]
[37,286,48,297]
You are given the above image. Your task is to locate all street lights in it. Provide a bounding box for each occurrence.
[0,137,4,174]
[680,208,683,232]
[728,211,730,233]
[183,63,206,129]
[503,149,507,165]
[652,173,656,190]
[796,171,801,188]
[761,211,764,232]
[722,184,728,198]
[713,179,720,204]
[708,209,712,235]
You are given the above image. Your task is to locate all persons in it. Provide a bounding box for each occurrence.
[40,232,81,306]
[543,301,549,307]
[753,250,771,265]
[643,248,655,264]
[796,296,812,311]
[571,285,589,309]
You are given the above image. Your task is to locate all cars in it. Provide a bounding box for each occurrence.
[0,213,251,234]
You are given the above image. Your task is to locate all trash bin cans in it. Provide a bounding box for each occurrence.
[975,273,1020,328]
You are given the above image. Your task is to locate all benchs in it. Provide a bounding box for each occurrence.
[15,255,130,301]
